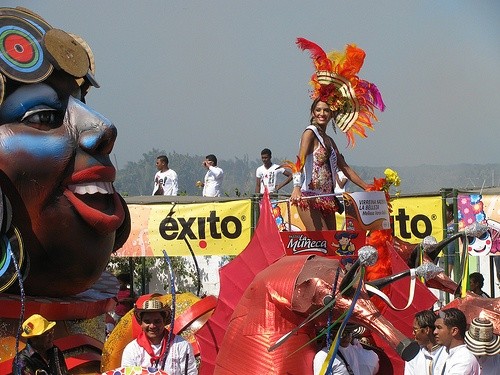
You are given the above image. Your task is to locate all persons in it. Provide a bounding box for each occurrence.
[330,231,358,258]
[120,299,199,375]
[469,272,491,298]
[0,5,125,300]
[11,314,70,375]
[341,256,357,278]
[403,309,446,375]
[151,155,178,196]
[195,154,224,198]
[255,148,293,193]
[115,273,136,317]
[312,323,380,375]
[429,308,482,375]
[288,96,375,232]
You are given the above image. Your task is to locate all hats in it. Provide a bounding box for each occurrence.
[21,314,56,338]
[134,300,171,326]
[464,317,500,355]
